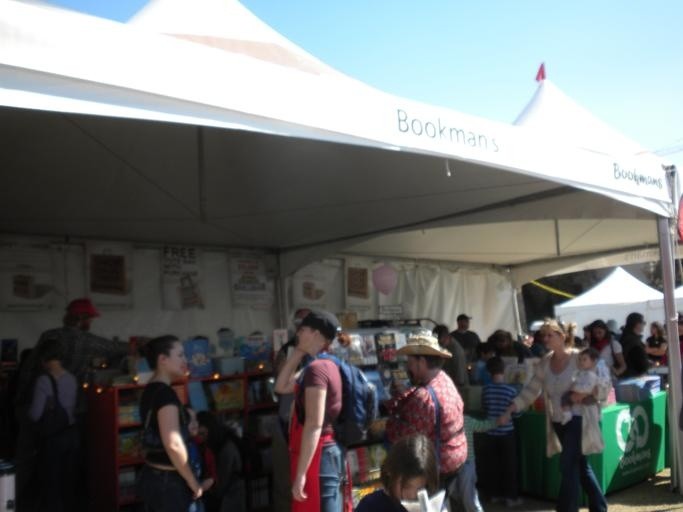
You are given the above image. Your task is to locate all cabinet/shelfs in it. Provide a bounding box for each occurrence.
[336,427,390,512]
[89,368,278,512]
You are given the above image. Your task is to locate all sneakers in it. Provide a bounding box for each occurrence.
[491,496,523,508]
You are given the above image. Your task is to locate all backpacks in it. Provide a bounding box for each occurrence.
[298,354,378,448]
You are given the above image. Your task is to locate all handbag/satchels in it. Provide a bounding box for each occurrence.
[143,424,189,448]
[38,404,74,463]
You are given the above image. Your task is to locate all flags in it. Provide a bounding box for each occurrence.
[535,61,547,82]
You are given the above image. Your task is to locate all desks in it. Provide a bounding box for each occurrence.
[519,390,666,509]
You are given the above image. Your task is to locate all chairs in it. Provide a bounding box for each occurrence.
[196,411,275,512]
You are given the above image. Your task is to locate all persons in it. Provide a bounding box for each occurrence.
[24,299,683,512]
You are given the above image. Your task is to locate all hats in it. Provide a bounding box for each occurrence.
[605,320,623,335]
[396,332,453,359]
[301,310,340,340]
[69,298,102,317]
[457,314,472,320]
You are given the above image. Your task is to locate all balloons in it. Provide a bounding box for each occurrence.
[371,266,398,296]
